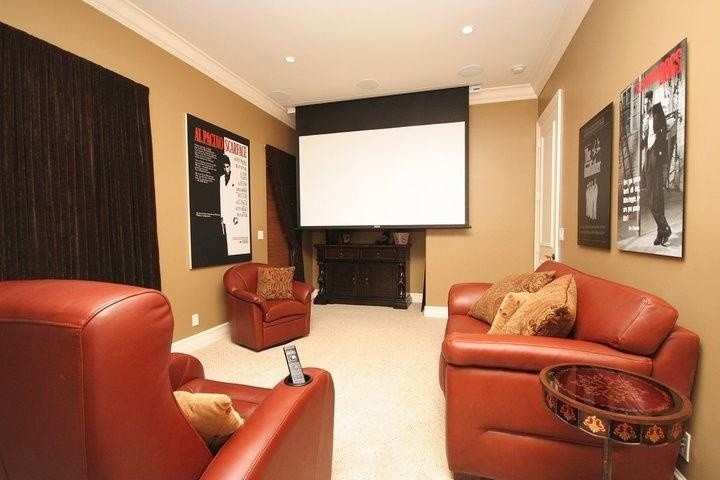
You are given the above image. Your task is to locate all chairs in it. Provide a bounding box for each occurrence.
[0,280,333,480]
[223,262,315,351]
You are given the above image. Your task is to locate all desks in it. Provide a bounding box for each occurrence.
[538,363,693,480]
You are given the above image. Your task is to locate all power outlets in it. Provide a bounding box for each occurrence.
[679,431,690,463]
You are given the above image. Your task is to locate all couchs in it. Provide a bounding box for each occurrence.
[438,259,699,480]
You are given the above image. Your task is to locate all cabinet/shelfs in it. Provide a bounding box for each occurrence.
[313,243,412,309]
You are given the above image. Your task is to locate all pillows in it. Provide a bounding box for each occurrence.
[170,390,245,450]
[534,260,678,355]
[467,270,577,337]
[257,266,296,300]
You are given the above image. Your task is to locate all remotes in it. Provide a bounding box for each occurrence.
[283,345,306,384]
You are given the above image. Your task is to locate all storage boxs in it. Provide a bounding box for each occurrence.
[393,232,409,245]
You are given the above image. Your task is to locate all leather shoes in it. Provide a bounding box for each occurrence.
[654,226,671,245]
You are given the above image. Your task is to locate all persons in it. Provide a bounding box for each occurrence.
[641,91,672,245]
[585,139,601,220]
[219,155,239,236]
